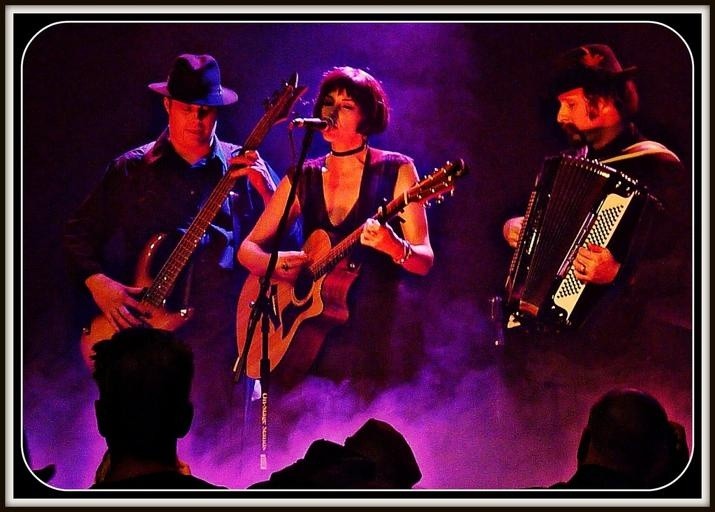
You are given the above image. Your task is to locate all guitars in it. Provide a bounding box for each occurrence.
[81,76,307,377]
[235,158,464,379]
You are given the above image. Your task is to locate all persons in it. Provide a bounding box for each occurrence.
[27,326,422,489]
[65,53,302,333]
[235,65,434,384]
[501,42,691,302]
[524,387,689,488]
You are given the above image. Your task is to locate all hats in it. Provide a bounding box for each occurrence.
[147,51,237,111]
[539,39,640,96]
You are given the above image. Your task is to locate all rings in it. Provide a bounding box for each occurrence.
[579,264,585,273]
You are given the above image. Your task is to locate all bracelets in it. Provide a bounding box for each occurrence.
[393,240,413,265]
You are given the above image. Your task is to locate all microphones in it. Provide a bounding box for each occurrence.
[294,117,334,132]
